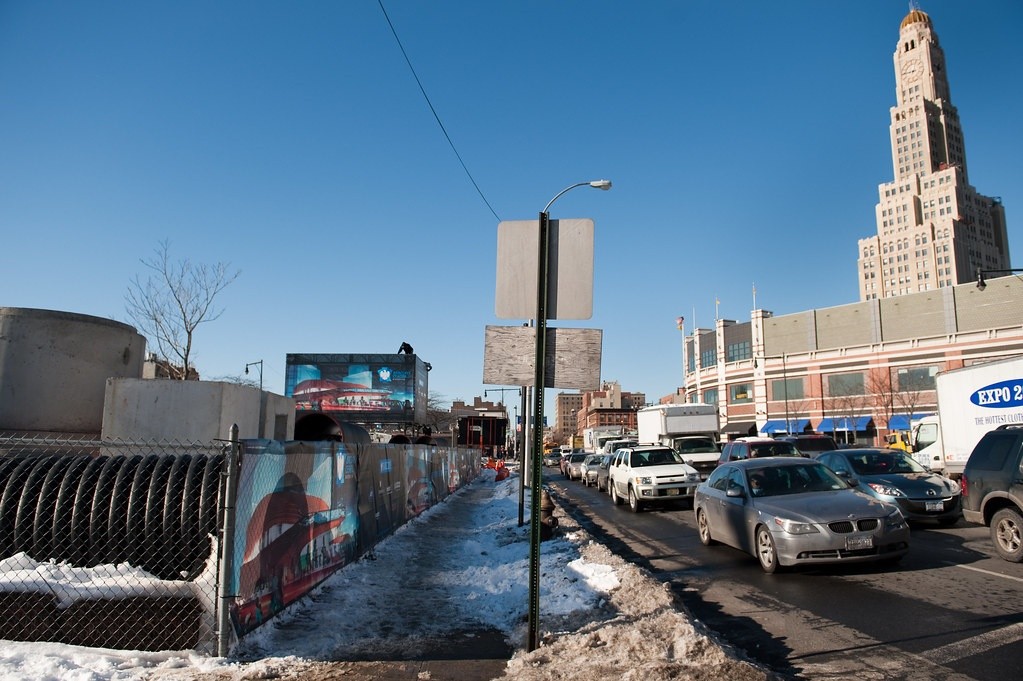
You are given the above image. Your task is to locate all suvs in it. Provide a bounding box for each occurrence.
[606,441,701,514]
[958,422,1023,564]
[718,436,806,465]
[774,433,839,459]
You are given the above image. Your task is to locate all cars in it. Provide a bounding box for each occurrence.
[799,447,963,529]
[542,424,639,492]
[692,454,912,576]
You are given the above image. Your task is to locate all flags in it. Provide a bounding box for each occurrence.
[675,316,684,330]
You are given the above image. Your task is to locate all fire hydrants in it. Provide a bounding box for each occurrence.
[540,488,559,542]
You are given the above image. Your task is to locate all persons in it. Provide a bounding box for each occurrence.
[343,396,367,406]
[750,474,773,496]
[300,398,320,410]
[500,446,514,460]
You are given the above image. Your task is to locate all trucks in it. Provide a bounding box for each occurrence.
[636,401,723,482]
[908,354,1023,480]
[883,430,913,453]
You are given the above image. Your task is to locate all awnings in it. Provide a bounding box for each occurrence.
[816,416,872,432]
[720,422,756,434]
[761,419,810,433]
[887,414,936,430]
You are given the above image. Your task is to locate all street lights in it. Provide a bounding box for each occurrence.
[244,359,264,390]
[753,353,790,435]
[525,178,615,656]
[483,387,522,461]
